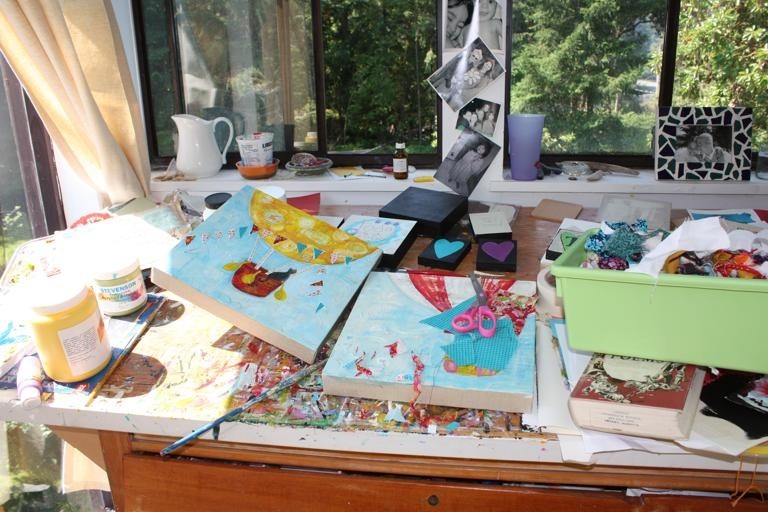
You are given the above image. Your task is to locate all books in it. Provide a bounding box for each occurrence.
[569,353,707,440]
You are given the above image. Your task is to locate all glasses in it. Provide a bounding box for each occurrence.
[472,54,478,62]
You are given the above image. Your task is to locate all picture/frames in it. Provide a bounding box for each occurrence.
[654,105,753,180]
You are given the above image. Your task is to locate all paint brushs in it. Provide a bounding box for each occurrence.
[83,297,167,408]
[160,357,330,456]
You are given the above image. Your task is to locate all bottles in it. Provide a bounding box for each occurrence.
[24,275,113,383]
[393,143,408,179]
[203,193,231,220]
[93,247,147,316]
[256,185,287,202]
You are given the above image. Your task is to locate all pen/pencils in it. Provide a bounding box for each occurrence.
[344,173,352,177]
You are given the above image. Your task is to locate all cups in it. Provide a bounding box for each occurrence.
[507,114,544,181]
[235,132,274,166]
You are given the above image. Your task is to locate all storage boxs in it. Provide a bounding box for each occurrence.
[550,228,768,378]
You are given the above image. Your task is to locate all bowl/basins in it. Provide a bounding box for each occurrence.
[235,159,280,179]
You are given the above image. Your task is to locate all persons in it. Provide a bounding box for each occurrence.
[432,48,495,107]
[447,142,491,190]
[462,0,503,49]
[675,134,722,164]
[697,133,731,163]
[458,104,495,136]
[445,0,474,48]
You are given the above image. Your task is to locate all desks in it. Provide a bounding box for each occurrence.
[0,202,768,512]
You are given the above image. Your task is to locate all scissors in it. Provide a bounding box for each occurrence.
[451,272,496,338]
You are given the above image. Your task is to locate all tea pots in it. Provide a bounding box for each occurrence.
[170,112,233,179]
